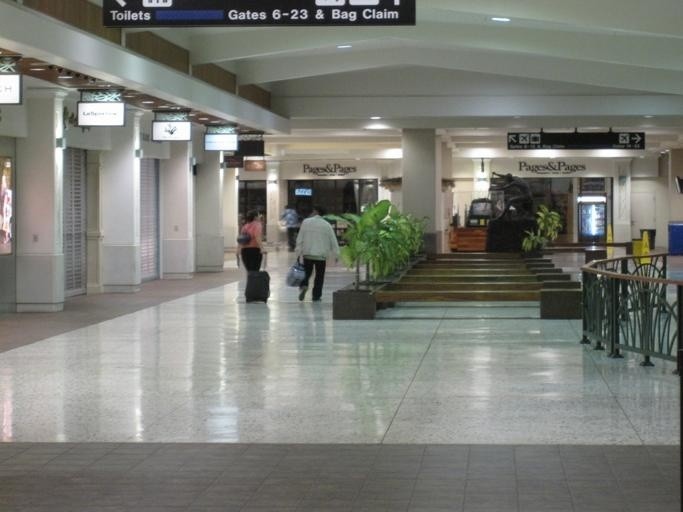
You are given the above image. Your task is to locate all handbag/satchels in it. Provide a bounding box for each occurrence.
[287,257,306,287]
[237,234,250,243]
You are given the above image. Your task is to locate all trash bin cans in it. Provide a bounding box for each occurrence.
[641,228,656,249]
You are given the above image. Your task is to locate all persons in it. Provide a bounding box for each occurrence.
[293,202,341,304]
[240,208,265,272]
[279,203,298,251]
[489,172,533,219]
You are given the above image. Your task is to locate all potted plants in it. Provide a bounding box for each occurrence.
[315,200,430,319]
[521,203,564,258]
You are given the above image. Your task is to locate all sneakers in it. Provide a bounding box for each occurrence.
[299,288,307,300]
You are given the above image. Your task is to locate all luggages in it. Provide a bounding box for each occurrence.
[245,270,272,304]
